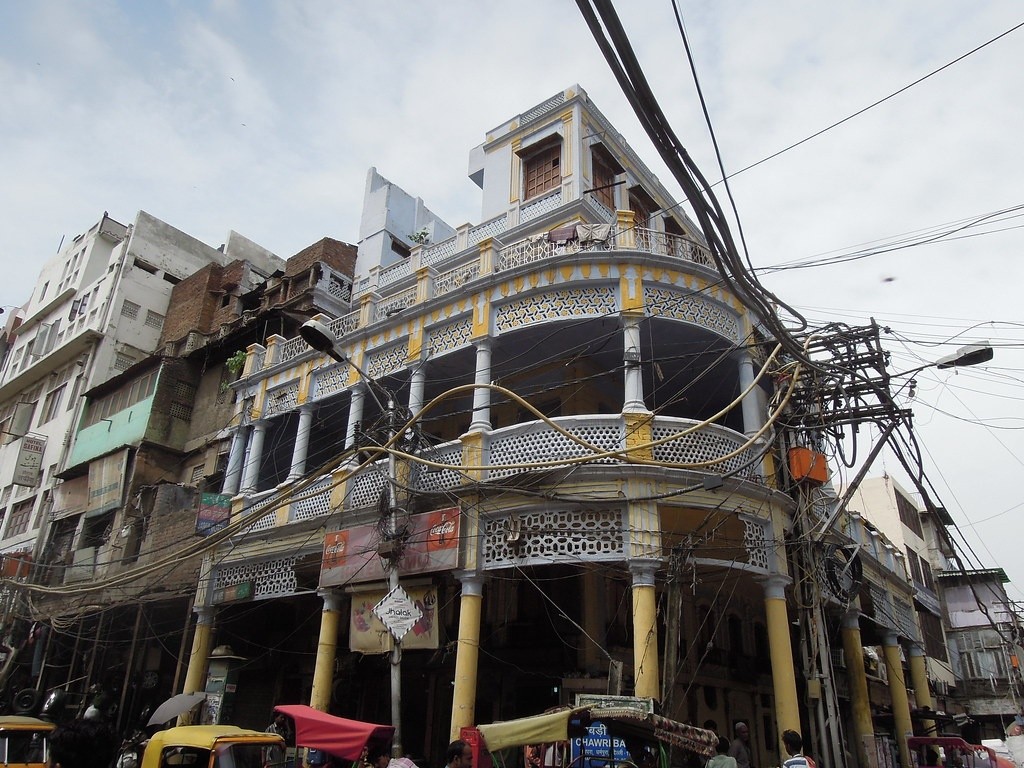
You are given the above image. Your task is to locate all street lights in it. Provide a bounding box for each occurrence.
[298,318,403,758]
[798,341,996,768]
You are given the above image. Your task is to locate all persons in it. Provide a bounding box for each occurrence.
[48,718,119,768]
[705,737,737,768]
[366,744,419,768]
[443,740,474,768]
[727,722,753,768]
[953,757,964,768]
[117,726,151,768]
[261,710,291,768]
[703,719,720,737]
[919,749,944,768]
[781,729,816,768]
